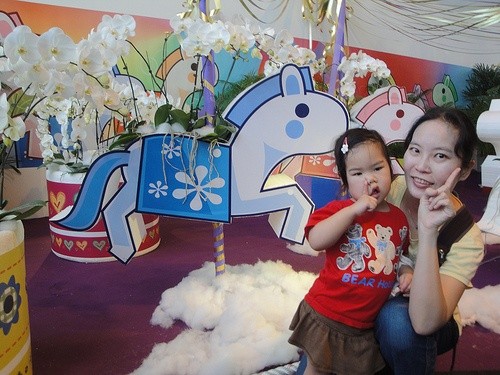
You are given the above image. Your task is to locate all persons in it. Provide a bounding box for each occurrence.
[294,107,485,375]
[287,128,414,375]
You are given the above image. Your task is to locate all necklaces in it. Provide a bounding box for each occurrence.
[405,204,418,230]
